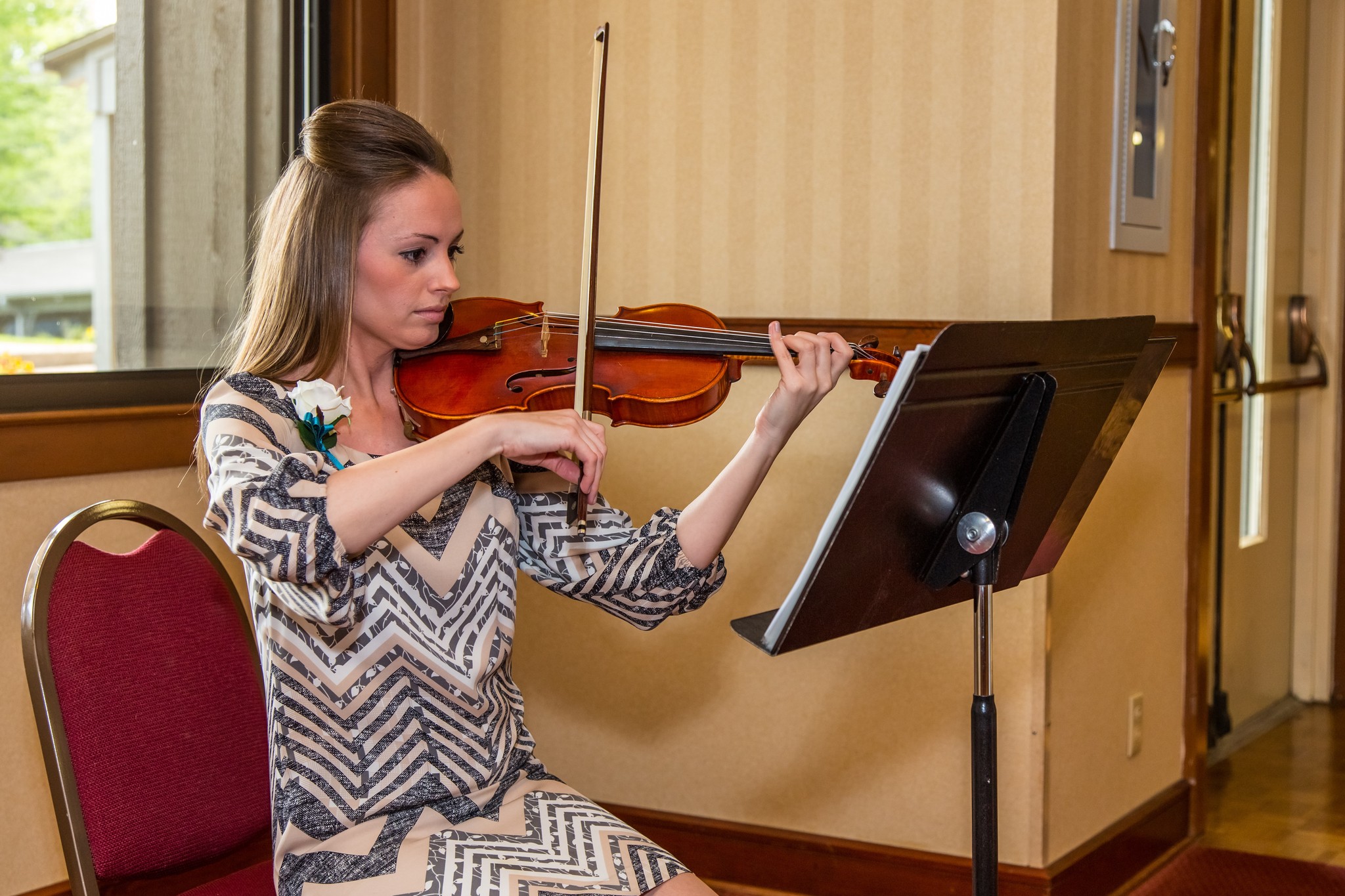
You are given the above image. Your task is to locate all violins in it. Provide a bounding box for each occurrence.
[390,297,905,444]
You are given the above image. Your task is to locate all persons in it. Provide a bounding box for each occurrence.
[191,100,856,896]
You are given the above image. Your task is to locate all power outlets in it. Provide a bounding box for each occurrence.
[1127,693,1146,757]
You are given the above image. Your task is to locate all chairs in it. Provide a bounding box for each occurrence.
[21,500,277,896]
